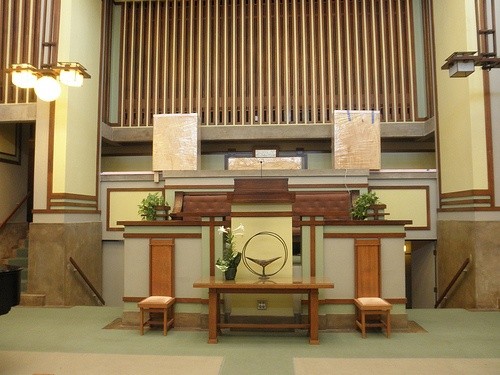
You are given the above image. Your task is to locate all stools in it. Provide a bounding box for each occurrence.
[138,295,177,335]
[354,297,393,337]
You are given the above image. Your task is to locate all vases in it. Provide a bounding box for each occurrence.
[226,264,239,280]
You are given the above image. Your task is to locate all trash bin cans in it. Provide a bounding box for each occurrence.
[0,266,22,315]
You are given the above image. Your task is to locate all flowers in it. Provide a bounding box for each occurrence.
[213,225,245,272]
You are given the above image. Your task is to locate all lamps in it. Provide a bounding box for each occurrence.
[5,42,90,101]
[441,51,500,77]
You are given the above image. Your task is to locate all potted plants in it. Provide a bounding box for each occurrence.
[137,193,171,222]
[351,191,385,220]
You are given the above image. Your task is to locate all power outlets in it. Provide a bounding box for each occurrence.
[257,298,268,310]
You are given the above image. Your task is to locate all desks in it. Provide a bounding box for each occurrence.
[193,278,334,345]
